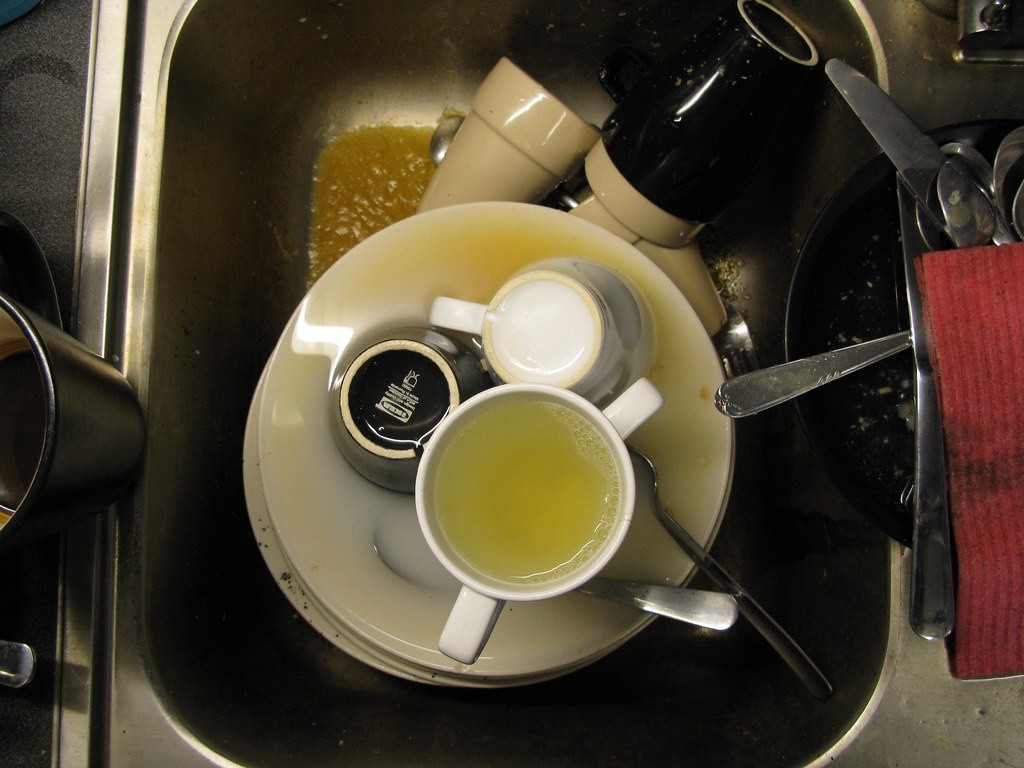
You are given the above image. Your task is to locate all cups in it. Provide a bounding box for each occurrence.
[584,1,818,247]
[413,386,634,665]
[329,324,496,491]
[1,293,146,549]
[428,257,655,394]
[568,195,728,336]
[415,59,597,215]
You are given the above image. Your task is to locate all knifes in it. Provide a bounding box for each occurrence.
[622,441,835,702]
[890,171,953,640]
[825,60,950,239]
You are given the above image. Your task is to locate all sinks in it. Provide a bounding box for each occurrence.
[133,2,914,768]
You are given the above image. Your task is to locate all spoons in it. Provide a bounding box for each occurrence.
[913,120,1022,247]
[373,511,738,636]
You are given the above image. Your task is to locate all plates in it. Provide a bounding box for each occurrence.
[785,119,1024,547]
[242,198,736,689]
